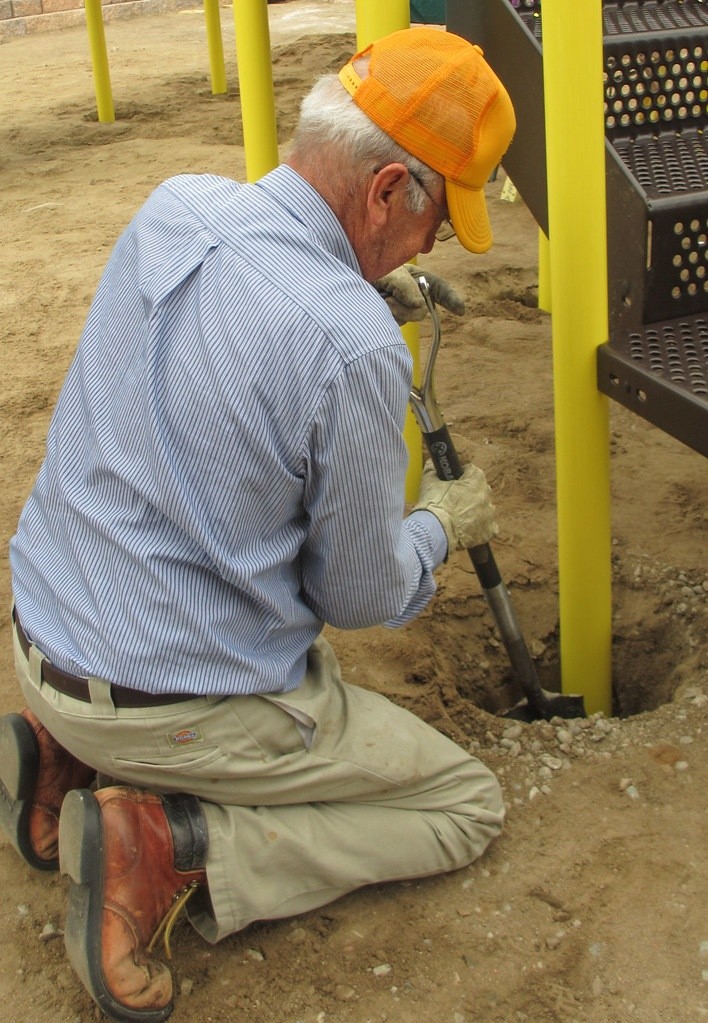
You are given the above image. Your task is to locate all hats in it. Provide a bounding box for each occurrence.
[337,26,518,255]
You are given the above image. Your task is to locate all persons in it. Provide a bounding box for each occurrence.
[0,29,518,1023]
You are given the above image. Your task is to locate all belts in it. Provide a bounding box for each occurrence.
[12,607,205,710]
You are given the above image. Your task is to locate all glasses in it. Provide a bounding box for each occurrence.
[371,164,456,242]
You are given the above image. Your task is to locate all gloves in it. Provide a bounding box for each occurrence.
[369,262,467,328]
[406,457,500,565]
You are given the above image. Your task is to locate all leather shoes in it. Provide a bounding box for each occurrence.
[0,706,99,872]
[55,784,211,1023]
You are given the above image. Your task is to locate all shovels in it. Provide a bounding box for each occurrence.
[378,276,585,722]
